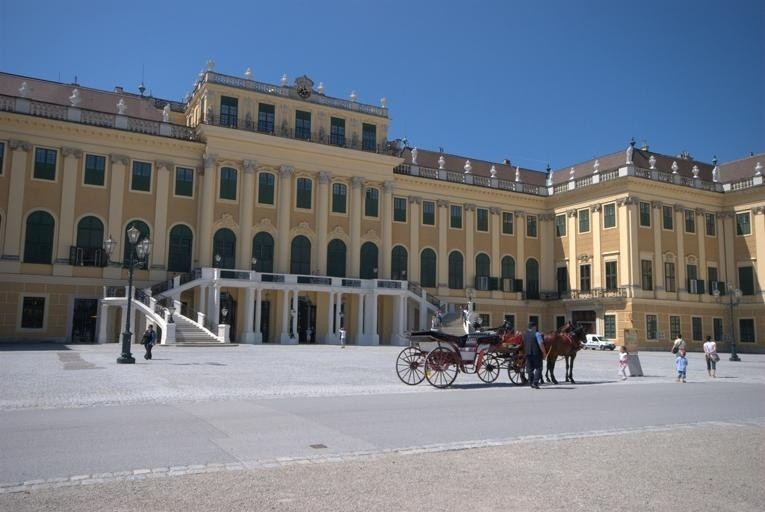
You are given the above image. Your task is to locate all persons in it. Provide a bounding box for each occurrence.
[525,321,547,390]
[676,349,687,383]
[338,327,346,349]
[140,324,157,361]
[670,335,686,356]
[702,335,719,377]
[618,346,629,380]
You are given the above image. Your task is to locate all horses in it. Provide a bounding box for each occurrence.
[540,320,588,383]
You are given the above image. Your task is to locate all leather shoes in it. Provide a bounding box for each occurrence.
[529,382,540,388]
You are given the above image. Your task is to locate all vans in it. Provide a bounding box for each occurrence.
[582,333,615,350]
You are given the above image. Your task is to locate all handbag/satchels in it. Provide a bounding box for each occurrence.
[672,348,678,354]
[710,354,720,362]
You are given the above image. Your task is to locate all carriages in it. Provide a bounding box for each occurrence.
[395,322,588,388]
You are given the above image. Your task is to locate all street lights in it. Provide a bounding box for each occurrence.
[713,280,743,361]
[103,225,153,363]
